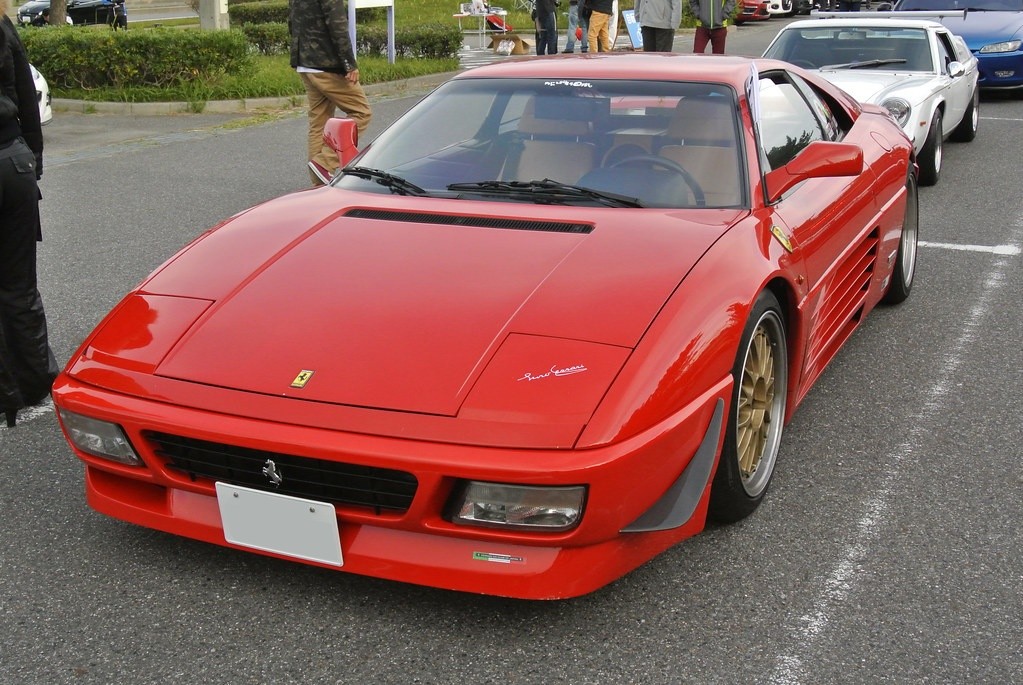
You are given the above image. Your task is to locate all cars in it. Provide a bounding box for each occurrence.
[760,0,794,17]
[16,0,114,27]
[730,0,771,26]
[875,0,1023,97]
[759,7,981,185]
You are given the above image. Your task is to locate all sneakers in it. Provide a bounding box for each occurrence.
[307,161,335,185]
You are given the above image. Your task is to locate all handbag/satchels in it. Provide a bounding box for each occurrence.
[496,37,515,55]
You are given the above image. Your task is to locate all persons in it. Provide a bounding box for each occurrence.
[530,0,562,56]
[26,7,49,28]
[1,0,62,430]
[65,12,73,26]
[633,0,682,53]
[563,0,613,53]
[688,0,736,56]
[104,0,128,30]
[838,0,862,12]
[287,0,372,187]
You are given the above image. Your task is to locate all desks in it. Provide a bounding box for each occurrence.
[452,11,508,55]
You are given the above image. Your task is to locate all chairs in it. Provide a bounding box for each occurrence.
[788,40,834,69]
[890,44,931,69]
[653,95,741,207]
[496,95,600,185]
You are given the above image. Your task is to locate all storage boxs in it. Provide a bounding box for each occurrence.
[490,32,536,55]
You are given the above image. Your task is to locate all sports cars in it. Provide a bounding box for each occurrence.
[50,50,920,604]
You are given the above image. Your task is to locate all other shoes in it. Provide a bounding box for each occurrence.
[582,50,587,53]
[562,50,573,53]
